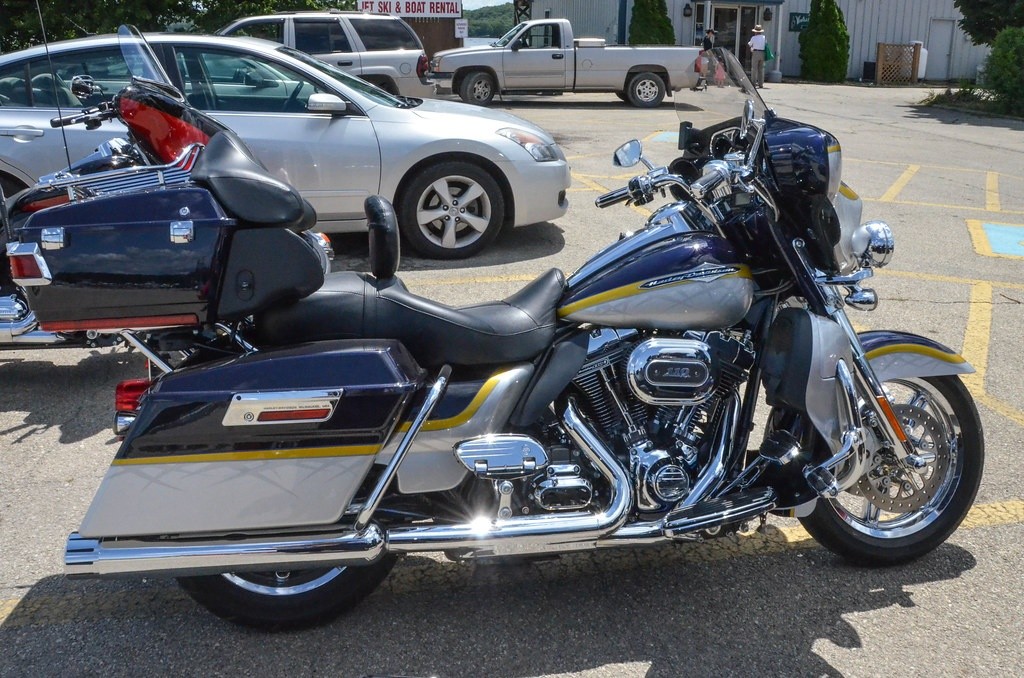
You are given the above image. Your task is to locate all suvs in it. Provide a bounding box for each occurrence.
[204,12,431,96]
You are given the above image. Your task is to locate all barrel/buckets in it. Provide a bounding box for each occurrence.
[900,40,928,79]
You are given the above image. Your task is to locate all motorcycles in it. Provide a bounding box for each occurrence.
[8,38,984,632]
[0,22,337,360]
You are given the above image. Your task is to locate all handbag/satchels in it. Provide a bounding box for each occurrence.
[764,44,774,61]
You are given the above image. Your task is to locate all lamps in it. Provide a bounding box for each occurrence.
[764,7,772,20]
[683,3,692,17]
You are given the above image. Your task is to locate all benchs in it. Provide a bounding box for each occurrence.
[0,72,85,107]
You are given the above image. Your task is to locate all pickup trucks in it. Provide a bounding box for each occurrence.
[430,18,708,107]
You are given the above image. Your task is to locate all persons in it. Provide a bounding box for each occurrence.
[704,29,717,84]
[714,56,725,88]
[748,25,767,88]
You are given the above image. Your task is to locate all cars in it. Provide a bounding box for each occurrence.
[0,31,572,260]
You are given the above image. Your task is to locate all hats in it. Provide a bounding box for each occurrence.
[705,28,715,34]
[752,25,765,33]
